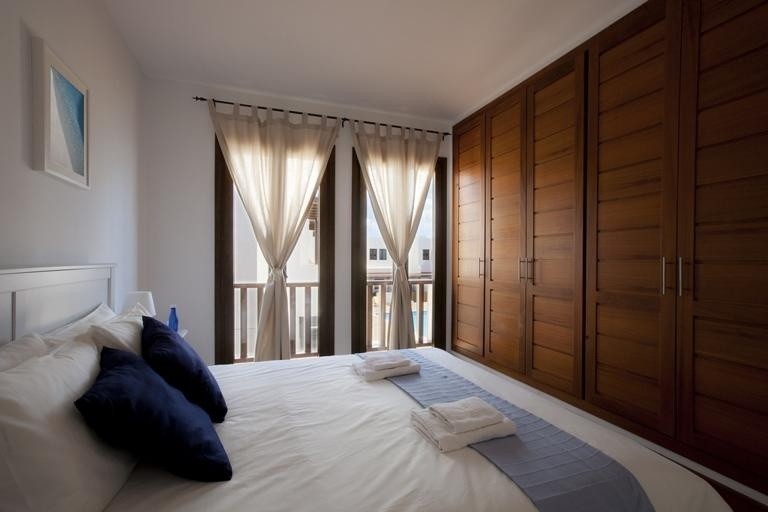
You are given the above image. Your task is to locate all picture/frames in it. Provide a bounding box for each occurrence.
[29,36,91,191]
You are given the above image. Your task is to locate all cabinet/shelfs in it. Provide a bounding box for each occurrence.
[585,2,768,478]
[448,109,485,364]
[485,42,584,414]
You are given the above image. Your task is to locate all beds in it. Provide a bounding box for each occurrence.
[0,261,733,512]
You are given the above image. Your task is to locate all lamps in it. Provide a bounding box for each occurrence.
[121,290,157,318]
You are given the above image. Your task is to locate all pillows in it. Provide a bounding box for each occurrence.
[2,302,233,511]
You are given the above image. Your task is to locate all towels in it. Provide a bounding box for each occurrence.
[352,350,420,383]
[408,396,518,454]
